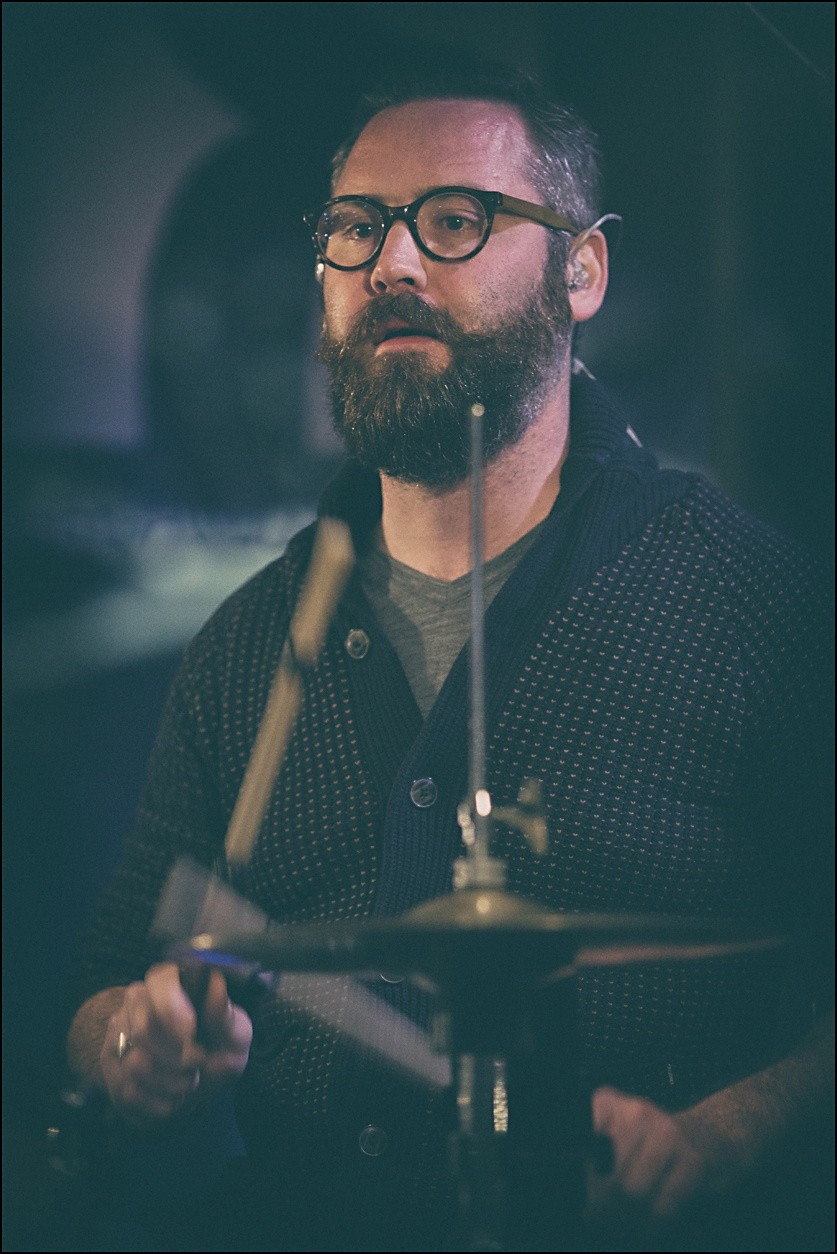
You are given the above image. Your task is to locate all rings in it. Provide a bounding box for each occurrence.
[114,1031,134,1061]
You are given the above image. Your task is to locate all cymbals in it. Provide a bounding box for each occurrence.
[191,886,790,968]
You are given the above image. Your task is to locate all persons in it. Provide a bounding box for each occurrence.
[0,59,836,1254]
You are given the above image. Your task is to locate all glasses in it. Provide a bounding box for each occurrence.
[303,185,584,273]
